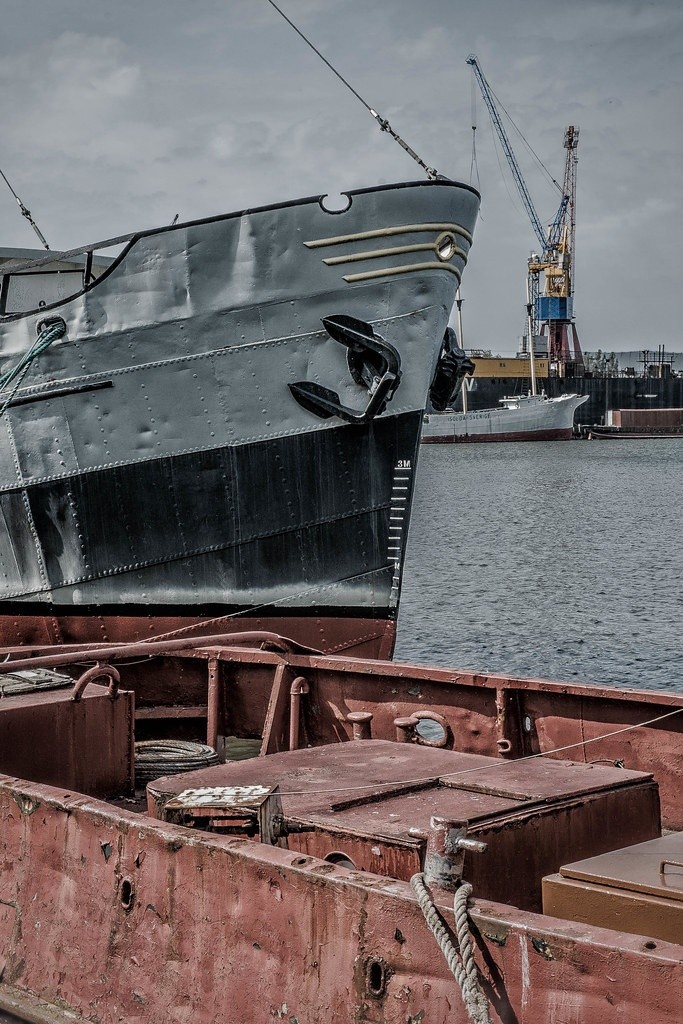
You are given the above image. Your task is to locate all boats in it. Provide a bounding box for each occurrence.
[407,393,586,441]
[0,186,480,659]
[574,406,683,439]
[0,643,683,1024]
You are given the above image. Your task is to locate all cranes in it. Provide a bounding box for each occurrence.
[464,50,559,254]
